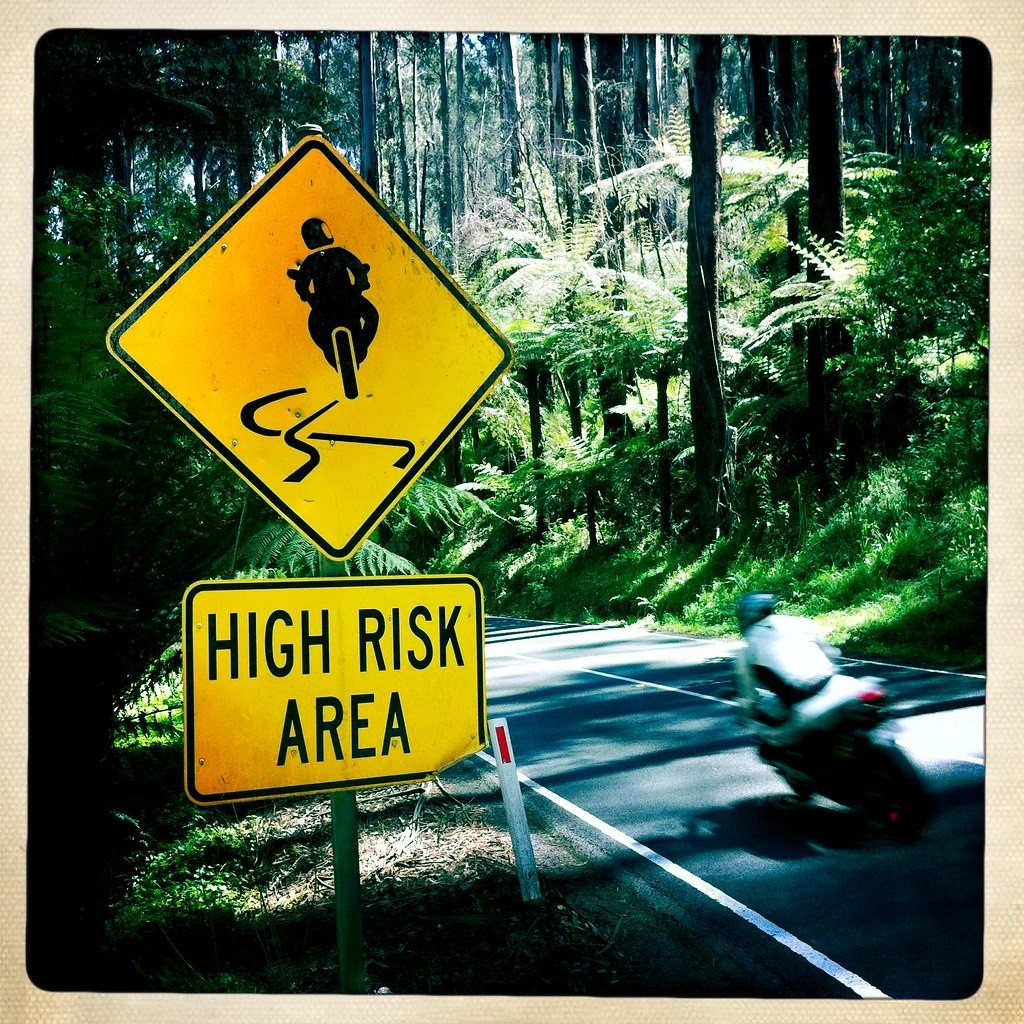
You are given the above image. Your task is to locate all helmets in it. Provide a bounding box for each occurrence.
[733,593,779,619]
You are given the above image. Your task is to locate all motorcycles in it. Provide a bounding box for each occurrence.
[732,651,926,837]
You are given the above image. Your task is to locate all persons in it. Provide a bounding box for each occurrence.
[733,593,866,729]
